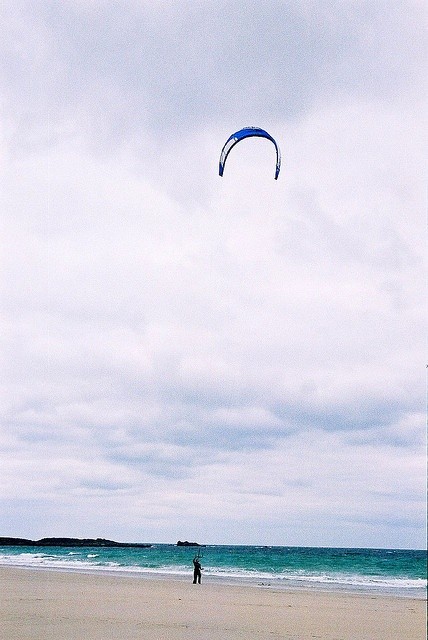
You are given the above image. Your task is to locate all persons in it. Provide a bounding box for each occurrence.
[193,556,204,584]
